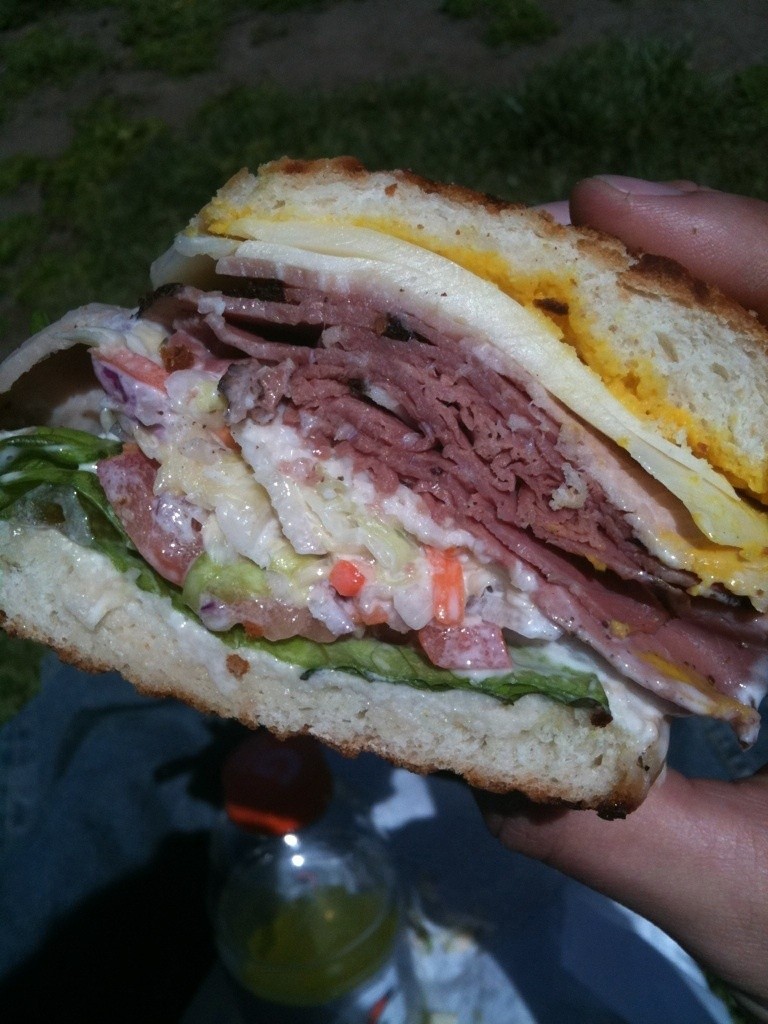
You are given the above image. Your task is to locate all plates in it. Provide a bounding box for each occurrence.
[0,648,768,1024]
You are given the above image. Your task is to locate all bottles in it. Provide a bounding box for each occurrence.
[211,719,533,1024]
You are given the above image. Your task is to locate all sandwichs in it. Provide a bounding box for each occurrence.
[0,155,766,818]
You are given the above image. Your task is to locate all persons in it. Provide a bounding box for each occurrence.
[469,174,768,1024]
